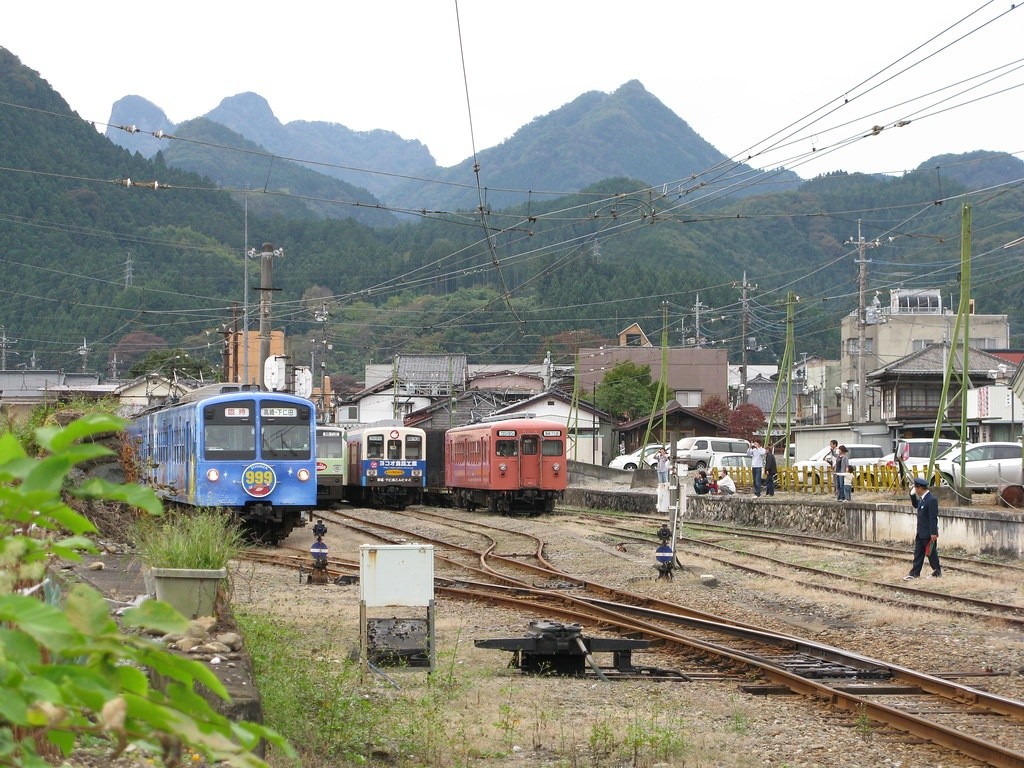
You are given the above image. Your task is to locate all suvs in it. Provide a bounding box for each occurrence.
[794,443,885,487]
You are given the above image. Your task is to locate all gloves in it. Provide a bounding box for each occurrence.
[910,488,916,495]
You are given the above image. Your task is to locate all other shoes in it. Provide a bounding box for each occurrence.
[838,499,843,502]
[927,570,942,579]
[903,573,920,581]
[764,494,773,497]
[843,499,849,502]
[753,496,759,498]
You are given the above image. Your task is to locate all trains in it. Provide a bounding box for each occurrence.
[426,417,568,515]
[346,420,427,507]
[129,381,319,544]
[315,427,351,508]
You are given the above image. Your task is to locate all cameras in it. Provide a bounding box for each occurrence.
[751,443,755,446]
[659,451,661,453]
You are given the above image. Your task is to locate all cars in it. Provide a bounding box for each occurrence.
[709,453,758,483]
[926,441,1023,493]
[875,452,897,484]
[893,437,986,486]
[783,443,798,464]
[608,440,669,470]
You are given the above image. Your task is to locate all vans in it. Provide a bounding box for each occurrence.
[666,437,752,471]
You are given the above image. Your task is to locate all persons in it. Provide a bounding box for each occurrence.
[694,471,710,495]
[717,470,735,495]
[747,438,766,498]
[763,446,777,497]
[902,478,942,580]
[653,447,668,483]
[823,439,854,502]
[706,479,718,495]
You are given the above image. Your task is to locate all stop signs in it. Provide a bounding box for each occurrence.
[310,542,329,562]
[656,545,673,563]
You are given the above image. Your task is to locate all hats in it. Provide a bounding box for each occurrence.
[914,478,929,487]
